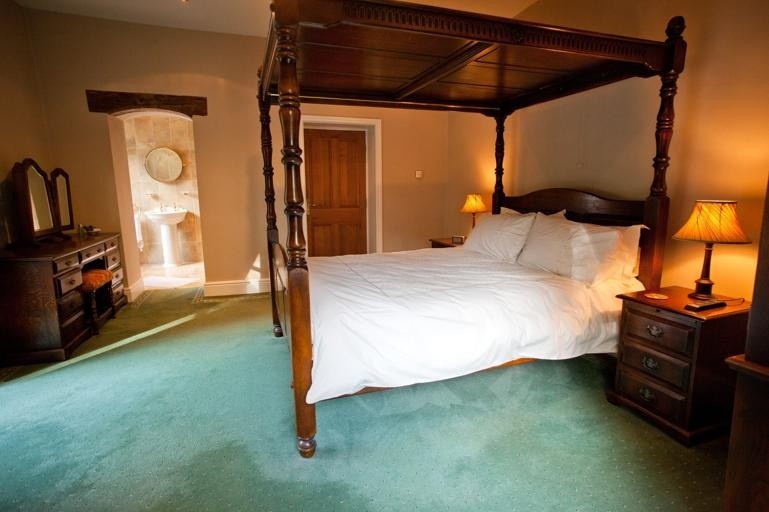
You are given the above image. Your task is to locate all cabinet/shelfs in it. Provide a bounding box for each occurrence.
[1,225,128,362]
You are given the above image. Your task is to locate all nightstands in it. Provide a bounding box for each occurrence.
[613,283,752,449]
[428,235,467,248]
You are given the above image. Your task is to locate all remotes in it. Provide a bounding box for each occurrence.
[684,298,726,312]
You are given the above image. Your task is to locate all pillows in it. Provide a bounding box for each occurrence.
[460,207,651,289]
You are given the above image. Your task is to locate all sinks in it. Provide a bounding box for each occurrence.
[145,207,186,225]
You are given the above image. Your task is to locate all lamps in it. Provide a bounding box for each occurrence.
[460,193,488,229]
[671,198,752,301]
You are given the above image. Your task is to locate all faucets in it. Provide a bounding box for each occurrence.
[173,202,177,211]
[160,204,163,213]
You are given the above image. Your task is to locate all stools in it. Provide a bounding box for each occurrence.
[81,268,116,335]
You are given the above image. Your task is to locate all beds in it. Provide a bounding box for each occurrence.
[265,187,672,458]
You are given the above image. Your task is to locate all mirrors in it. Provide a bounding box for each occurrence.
[143,146,184,184]
[11,157,75,238]
